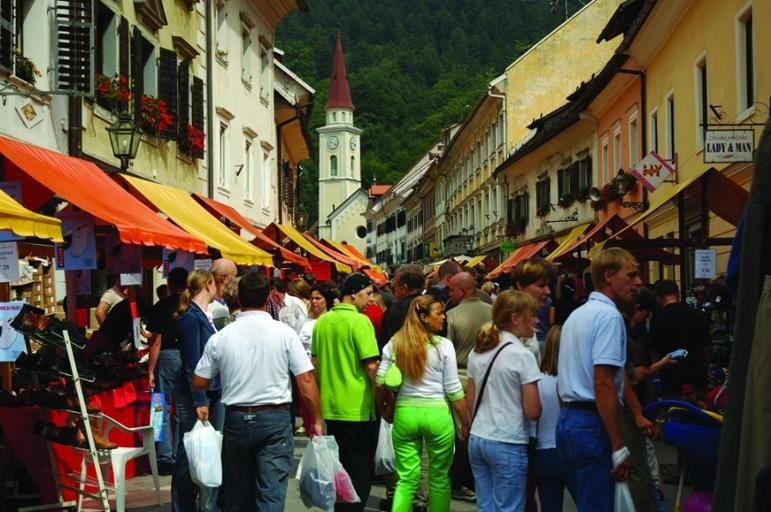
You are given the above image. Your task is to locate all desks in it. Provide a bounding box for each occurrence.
[1,369,174,503]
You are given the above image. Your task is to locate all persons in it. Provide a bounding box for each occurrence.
[141,247,710,511]
[95,274,128,327]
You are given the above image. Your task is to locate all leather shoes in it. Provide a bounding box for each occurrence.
[31,417,118,448]
[17,310,90,345]
[15,352,95,379]
[27,387,102,413]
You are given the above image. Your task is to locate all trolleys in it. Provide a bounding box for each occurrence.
[636,400,725,511]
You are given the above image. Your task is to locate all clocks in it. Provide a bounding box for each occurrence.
[327,135,339,150]
[350,137,358,151]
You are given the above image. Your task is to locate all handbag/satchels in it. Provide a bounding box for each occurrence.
[384,364,403,391]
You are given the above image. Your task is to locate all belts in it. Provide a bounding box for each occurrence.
[562,400,598,411]
[224,402,288,413]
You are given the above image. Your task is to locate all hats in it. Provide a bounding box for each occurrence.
[342,272,373,295]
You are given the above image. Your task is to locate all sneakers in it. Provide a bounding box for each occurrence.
[451,486,476,504]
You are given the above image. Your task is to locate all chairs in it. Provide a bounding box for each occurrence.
[40,405,164,512]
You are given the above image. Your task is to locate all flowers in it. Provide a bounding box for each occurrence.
[96,73,130,112]
[140,94,173,130]
[185,124,205,155]
[13,51,43,85]
